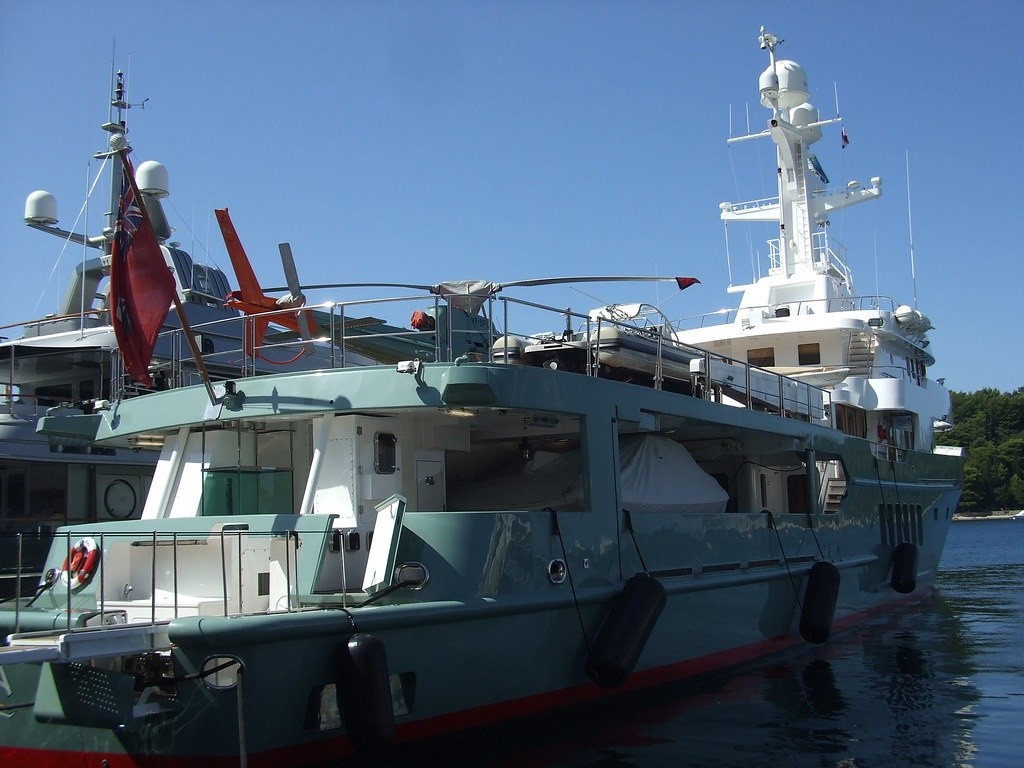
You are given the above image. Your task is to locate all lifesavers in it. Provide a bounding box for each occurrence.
[59,536,100,591]
[877,417,888,444]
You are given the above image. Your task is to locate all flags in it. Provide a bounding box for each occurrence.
[842,126,850,150]
[106,159,175,390]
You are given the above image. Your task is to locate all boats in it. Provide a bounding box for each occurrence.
[0,28,965,768]
